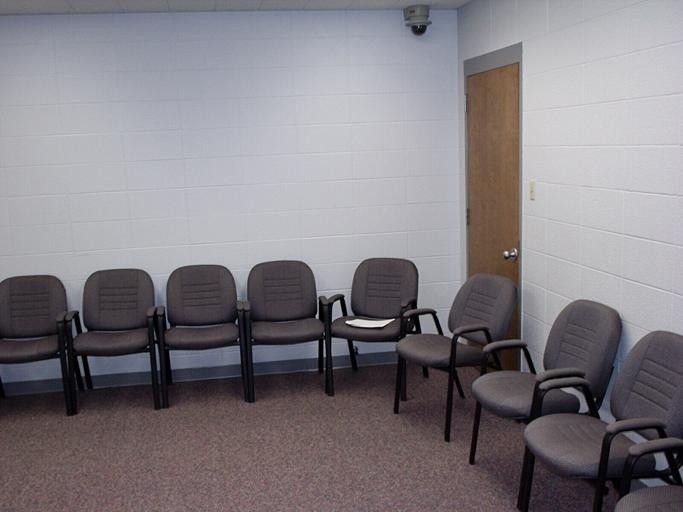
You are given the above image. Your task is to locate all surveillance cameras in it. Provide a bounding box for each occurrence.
[405,5,432,36]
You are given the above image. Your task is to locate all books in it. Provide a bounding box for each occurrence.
[345,318,395,328]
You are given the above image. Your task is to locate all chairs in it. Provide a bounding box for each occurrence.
[328,257,430,396]
[614,437,682,512]
[155,265,251,402]
[64,269,161,411]
[469,300,622,509]
[518,330,682,512]
[243,261,330,403]
[394,273,518,442]
[0,276,71,417]
